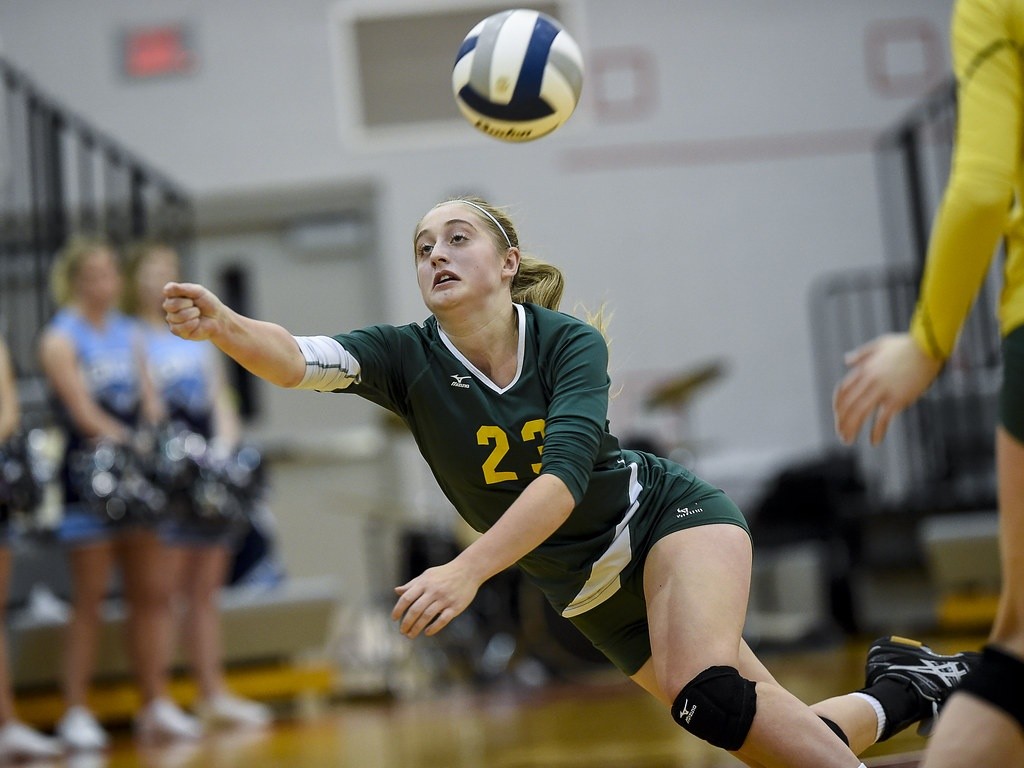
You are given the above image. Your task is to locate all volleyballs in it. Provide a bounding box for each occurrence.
[451,8,587,143]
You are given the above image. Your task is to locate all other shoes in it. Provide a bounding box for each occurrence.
[0,720,68,764]
[56,703,113,750]
[197,692,275,733]
[132,696,204,742]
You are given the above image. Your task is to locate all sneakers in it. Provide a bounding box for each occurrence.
[865,635,980,738]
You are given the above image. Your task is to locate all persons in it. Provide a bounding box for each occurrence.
[163,197,977,768]
[0,230,276,756]
[831,0,1024,768]
